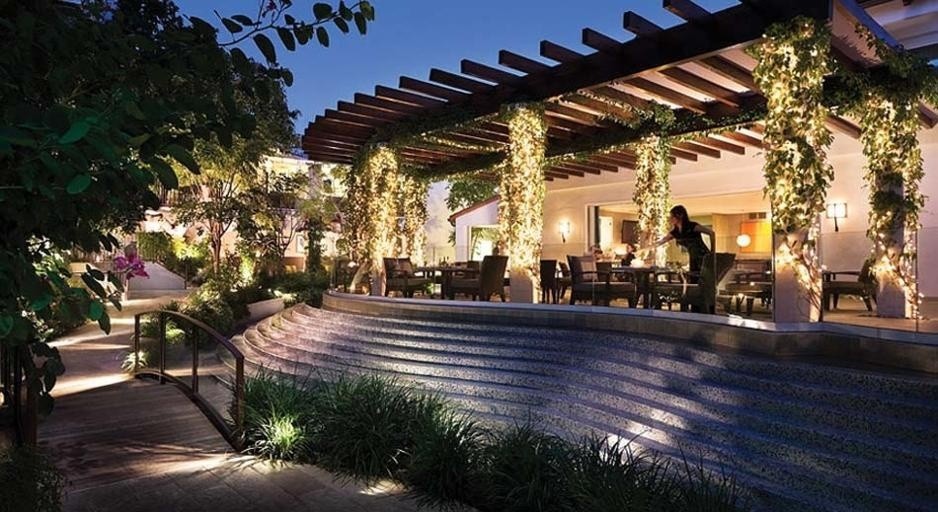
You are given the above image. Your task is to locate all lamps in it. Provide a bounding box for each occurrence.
[825,202,848,232]
[736,233,751,247]
[556,218,573,243]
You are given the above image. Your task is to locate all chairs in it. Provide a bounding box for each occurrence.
[381,251,879,322]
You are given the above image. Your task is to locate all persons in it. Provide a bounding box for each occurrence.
[619,242,636,266]
[633,205,716,314]
